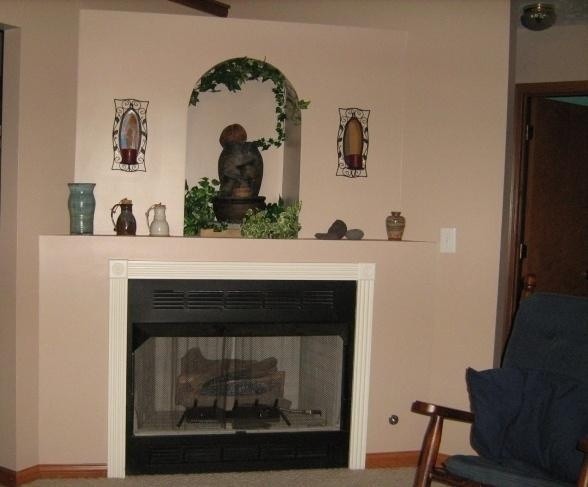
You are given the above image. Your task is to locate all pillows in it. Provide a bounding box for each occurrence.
[465,364,586,481]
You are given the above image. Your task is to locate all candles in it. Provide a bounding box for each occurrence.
[349,154,362,168]
[122,148,137,164]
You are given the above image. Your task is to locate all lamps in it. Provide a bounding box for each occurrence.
[521,4,556,30]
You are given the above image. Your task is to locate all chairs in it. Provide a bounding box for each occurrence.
[406,274,587,487]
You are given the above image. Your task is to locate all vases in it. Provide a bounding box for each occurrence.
[384,211,407,240]
[66,182,96,235]
